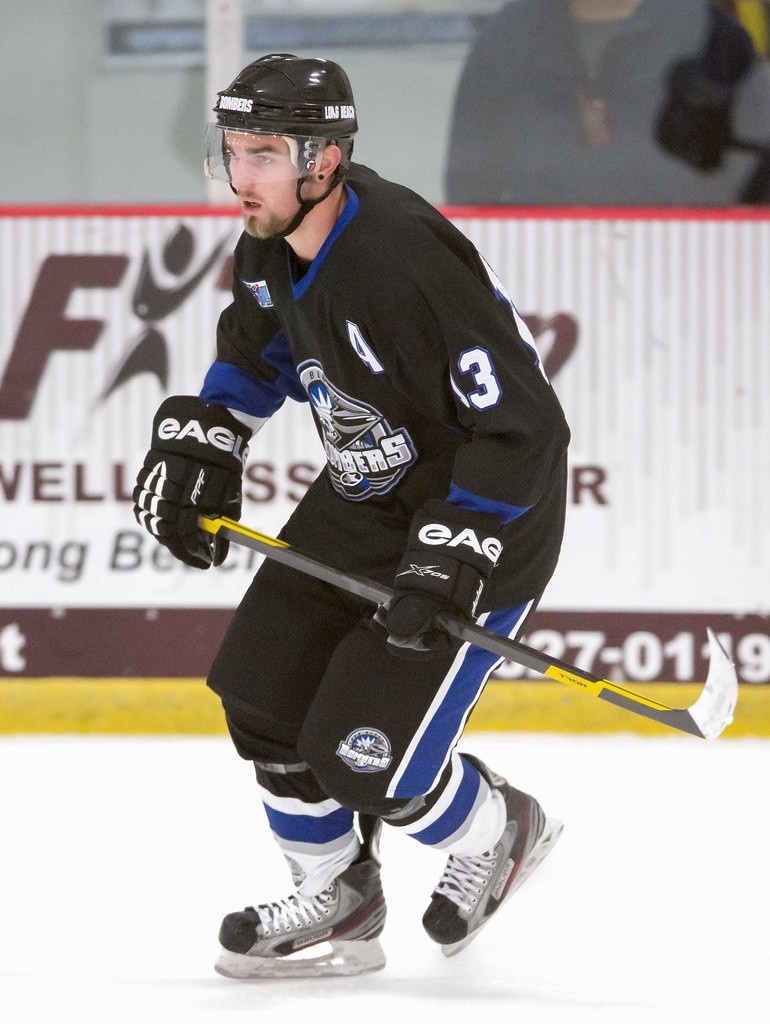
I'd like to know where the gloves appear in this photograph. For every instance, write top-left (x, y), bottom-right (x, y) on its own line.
top-left (132, 395), bottom-right (252, 568)
top-left (368, 500), bottom-right (508, 654)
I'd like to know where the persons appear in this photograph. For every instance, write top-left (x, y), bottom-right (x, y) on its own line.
top-left (446, 0), bottom-right (770, 204)
top-left (133, 54), bottom-right (570, 980)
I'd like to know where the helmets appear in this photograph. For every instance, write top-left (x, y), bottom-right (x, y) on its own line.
top-left (213, 54), bottom-right (359, 178)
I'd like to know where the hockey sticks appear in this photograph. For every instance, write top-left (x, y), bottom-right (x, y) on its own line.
top-left (196, 511), bottom-right (740, 742)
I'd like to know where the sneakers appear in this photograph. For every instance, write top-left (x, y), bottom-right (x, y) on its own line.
top-left (214, 858), bottom-right (386, 979)
top-left (422, 753), bottom-right (567, 957)
